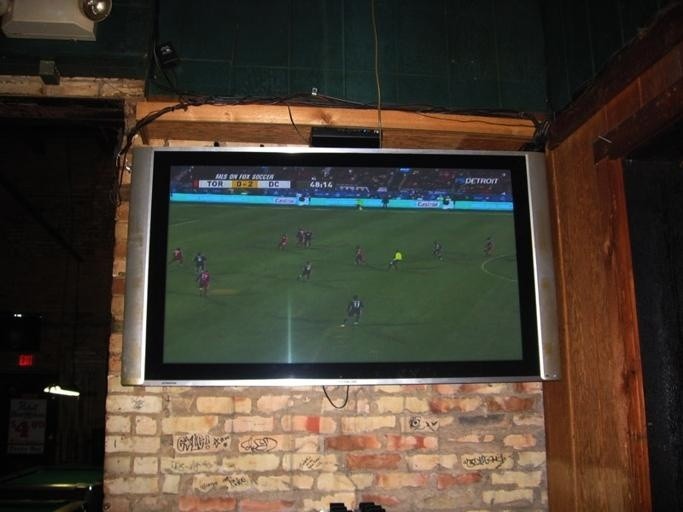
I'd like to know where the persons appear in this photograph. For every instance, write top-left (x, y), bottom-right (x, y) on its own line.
top-left (173, 247), bottom-right (184, 267)
top-left (352, 244), bottom-right (366, 265)
top-left (431, 239), bottom-right (446, 262)
top-left (480, 235), bottom-right (494, 256)
top-left (279, 231), bottom-right (288, 251)
top-left (190, 252), bottom-right (206, 273)
top-left (299, 260), bottom-right (312, 281)
top-left (192, 269), bottom-right (211, 297)
top-left (337, 293), bottom-right (364, 329)
top-left (387, 248), bottom-right (403, 272)
top-left (304, 227), bottom-right (312, 249)
top-left (294, 226), bottom-right (305, 248)
top-left (169, 166), bottom-right (513, 213)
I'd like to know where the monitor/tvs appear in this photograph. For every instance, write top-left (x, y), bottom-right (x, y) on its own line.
top-left (120, 145), bottom-right (564, 388)
